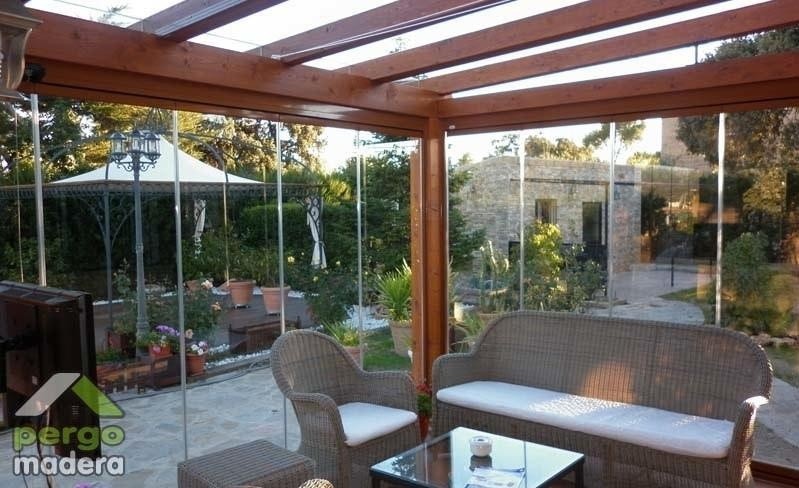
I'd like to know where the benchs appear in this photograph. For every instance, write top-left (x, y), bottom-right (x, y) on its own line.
top-left (428, 308), bottom-right (772, 486)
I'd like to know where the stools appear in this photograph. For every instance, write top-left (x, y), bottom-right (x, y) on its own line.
top-left (178, 438), bottom-right (315, 488)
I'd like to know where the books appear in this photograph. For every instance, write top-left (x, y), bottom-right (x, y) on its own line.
top-left (464, 466), bottom-right (527, 488)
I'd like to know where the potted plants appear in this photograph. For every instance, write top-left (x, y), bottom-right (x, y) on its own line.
top-left (374, 256), bottom-right (415, 357)
top-left (161, 223), bottom-right (292, 315)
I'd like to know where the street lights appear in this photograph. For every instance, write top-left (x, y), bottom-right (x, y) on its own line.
top-left (111, 127), bottom-right (160, 356)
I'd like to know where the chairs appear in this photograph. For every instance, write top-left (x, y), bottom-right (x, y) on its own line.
top-left (269, 329), bottom-right (419, 488)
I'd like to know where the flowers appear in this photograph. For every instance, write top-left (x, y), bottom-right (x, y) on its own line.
top-left (134, 324), bottom-right (211, 363)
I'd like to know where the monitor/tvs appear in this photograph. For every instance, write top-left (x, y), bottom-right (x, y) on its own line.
top-left (0, 276), bottom-right (104, 466)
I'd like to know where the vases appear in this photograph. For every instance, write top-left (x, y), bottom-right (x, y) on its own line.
top-left (184, 350), bottom-right (205, 376)
